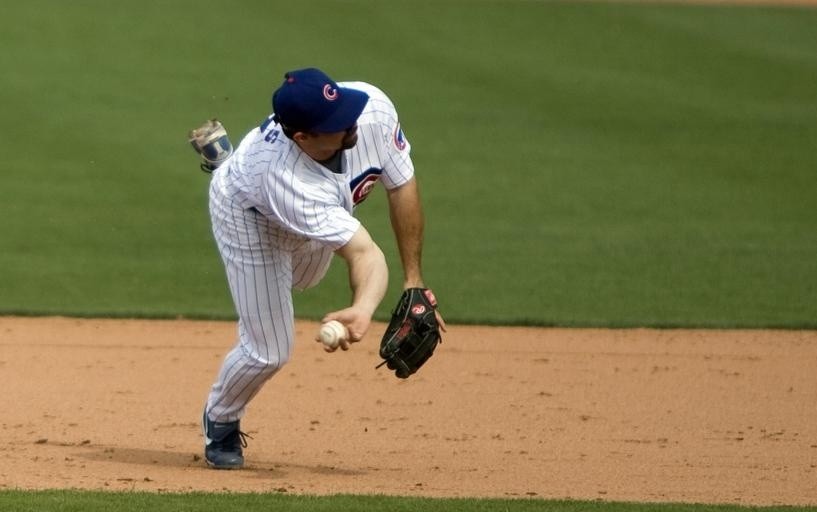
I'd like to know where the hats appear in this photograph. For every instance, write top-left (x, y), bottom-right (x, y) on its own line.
top-left (273, 67), bottom-right (369, 133)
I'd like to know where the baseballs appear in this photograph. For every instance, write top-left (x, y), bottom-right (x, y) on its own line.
top-left (319, 321), bottom-right (346, 348)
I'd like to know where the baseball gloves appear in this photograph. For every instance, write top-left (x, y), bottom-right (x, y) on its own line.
top-left (379, 289), bottom-right (442, 379)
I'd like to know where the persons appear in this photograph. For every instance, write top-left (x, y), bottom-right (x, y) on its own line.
top-left (187, 65), bottom-right (447, 471)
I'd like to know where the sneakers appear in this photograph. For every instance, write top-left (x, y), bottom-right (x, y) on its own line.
top-left (188, 120), bottom-right (234, 173)
top-left (202, 402), bottom-right (254, 469)
